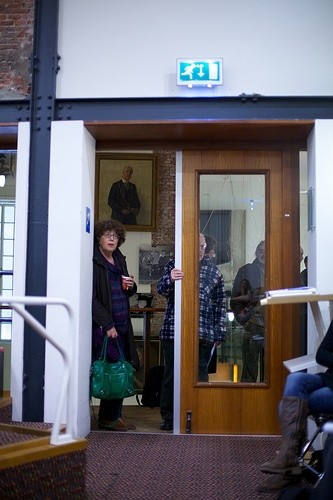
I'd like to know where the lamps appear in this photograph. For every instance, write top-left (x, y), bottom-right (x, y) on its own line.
top-left (0, 153), bottom-right (14, 187)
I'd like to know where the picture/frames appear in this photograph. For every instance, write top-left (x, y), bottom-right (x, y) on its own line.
top-left (133, 336), bottom-right (165, 394)
top-left (94, 152), bottom-right (158, 233)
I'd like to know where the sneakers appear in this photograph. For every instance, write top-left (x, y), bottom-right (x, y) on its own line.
top-left (120, 416), bottom-right (136, 430)
top-left (98, 417), bottom-right (129, 431)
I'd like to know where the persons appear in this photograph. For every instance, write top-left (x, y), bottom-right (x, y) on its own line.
top-left (155, 233), bottom-right (226, 433)
top-left (255, 319), bottom-right (332, 492)
top-left (107, 166), bottom-right (140, 224)
top-left (231, 240), bottom-right (266, 383)
top-left (92, 220), bottom-right (138, 431)
top-left (299, 244), bottom-right (309, 285)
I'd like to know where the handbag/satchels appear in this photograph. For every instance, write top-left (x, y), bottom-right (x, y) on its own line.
top-left (91, 334), bottom-right (135, 400)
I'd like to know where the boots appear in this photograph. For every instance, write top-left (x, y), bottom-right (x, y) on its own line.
top-left (256, 475), bottom-right (303, 492)
top-left (259, 397), bottom-right (310, 474)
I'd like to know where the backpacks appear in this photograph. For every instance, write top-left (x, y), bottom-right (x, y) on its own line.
top-left (141, 365), bottom-right (164, 406)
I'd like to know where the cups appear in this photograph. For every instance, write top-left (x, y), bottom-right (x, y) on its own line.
top-left (122, 277), bottom-right (132, 290)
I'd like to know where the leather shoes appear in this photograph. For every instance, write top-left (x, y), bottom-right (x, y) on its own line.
top-left (160, 415), bottom-right (173, 430)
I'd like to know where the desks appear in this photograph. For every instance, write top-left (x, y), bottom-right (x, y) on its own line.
top-left (130, 308), bottom-right (166, 385)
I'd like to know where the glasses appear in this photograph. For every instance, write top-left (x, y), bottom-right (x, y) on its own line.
top-left (199, 242), bottom-right (206, 250)
top-left (102, 233), bottom-right (119, 239)
